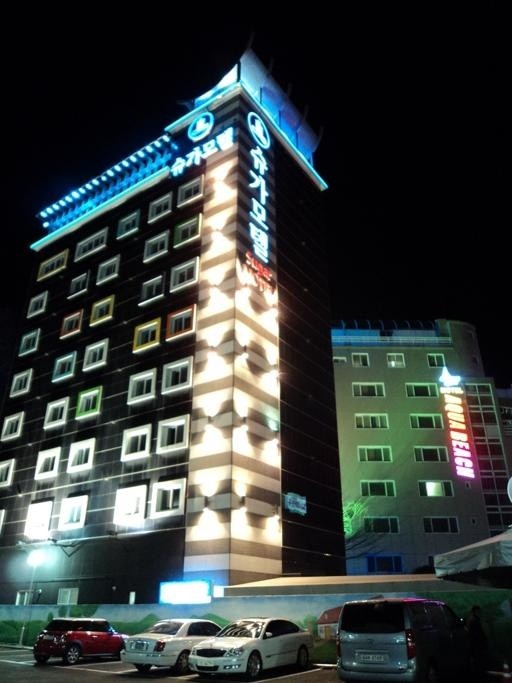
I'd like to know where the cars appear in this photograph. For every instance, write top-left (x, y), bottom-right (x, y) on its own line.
top-left (32, 615), bottom-right (128, 663)
top-left (188, 616), bottom-right (315, 681)
top-left (120, 617), bottom-right (223, 673)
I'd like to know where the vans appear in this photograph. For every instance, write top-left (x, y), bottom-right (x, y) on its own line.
top-left (334, 597), bottom-right (472, 682)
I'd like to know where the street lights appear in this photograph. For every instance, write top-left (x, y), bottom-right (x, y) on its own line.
top-left (16, 552), bottom-right (46, 648)
